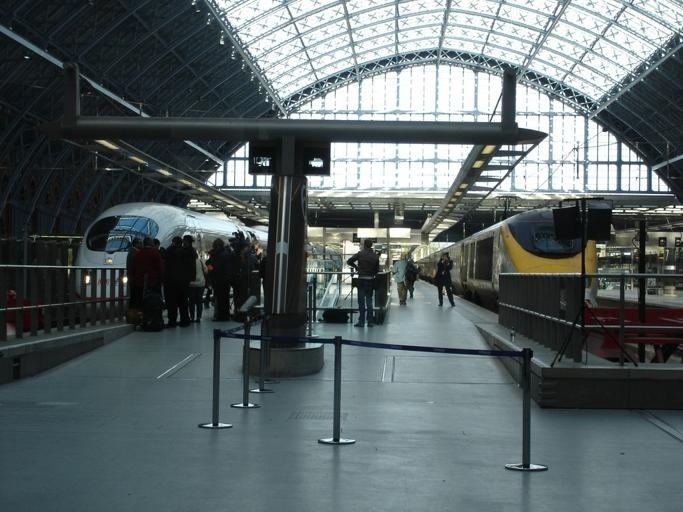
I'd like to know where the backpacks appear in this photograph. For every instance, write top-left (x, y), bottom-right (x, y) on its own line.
top-left (404, 260), bottom-right (417, 284)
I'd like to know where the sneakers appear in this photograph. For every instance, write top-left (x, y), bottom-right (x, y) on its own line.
top-left (400, 294), bottom-right (414, 306)
top-left (366, 324), bottom-right (373, 328)
top-left (353, 323), bottom-right (364, 327)
top-left (166, 317), bottom-right (201, 329)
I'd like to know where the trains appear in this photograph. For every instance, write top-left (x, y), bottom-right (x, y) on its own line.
top-left (74, 201), bottom-right (341, 315)
top-left (415, 206), bottom-right (598, 314)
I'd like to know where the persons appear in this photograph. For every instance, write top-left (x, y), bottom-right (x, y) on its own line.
top-left (346, 238), bottom-right (379, 327)
top-left (405, 257), bottom-right (416, 299)
top-left (432, 251), bottom-right (455, 307)
top-left (389, 252), bottom-right (418, 306)
top-left (126, 232), bottom-right (260, 333)
